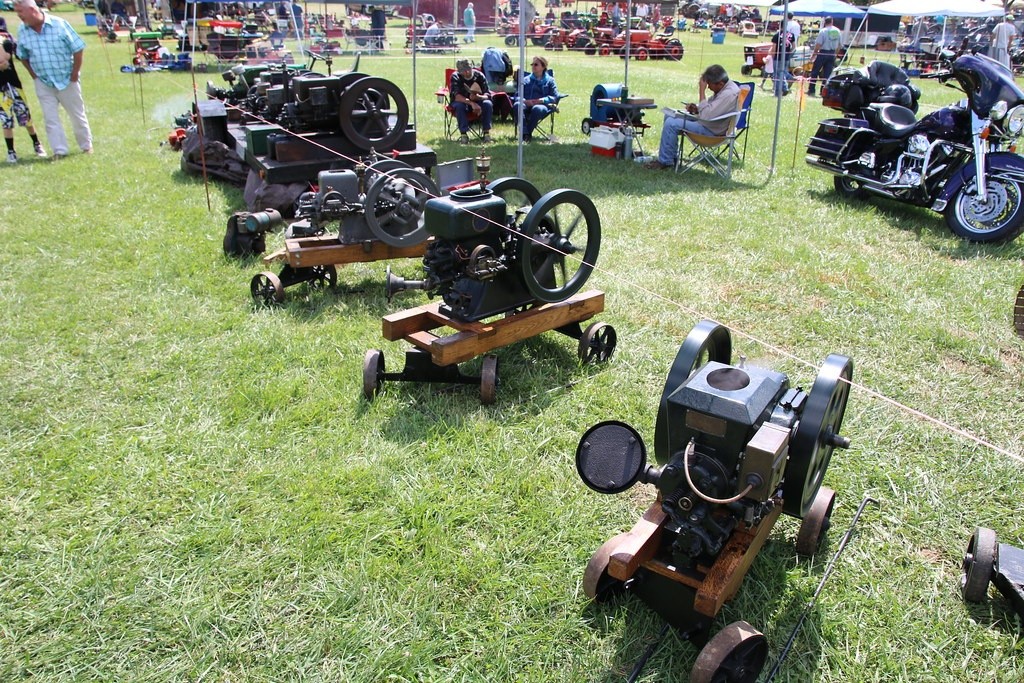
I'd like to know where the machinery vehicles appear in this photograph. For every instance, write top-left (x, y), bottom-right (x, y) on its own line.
top-left (504, 10), bottom-right (684, 61)
top-left (681, 0), bottom-right (723, 20)
top-left (741, 39), bottom-right (777, 78)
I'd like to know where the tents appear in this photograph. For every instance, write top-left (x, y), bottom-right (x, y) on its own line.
top-left (864, 0), bottom-right (1006, 73)
top-left (762, 0), bottom-right (868, 69)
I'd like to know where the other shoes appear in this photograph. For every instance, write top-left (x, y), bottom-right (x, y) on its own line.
top-left (8, 152), bottom-right (16, 163)
top-left (483, 136), bottom-right (493, 143)
top-left (523, 133), bottom-right (532, 144)
top-left (788, 77), bottom-right (796, 87)
top-left (804, 91), bottom-right (815, 96)
top-left (34, 143), bottom-right (46, 158)
top-left (53, 153), bottom-right (64, 160)
top-left (463, 38), bottom-right (468, 43)
top-left (783, 90), bottom-right (789, 95)
top-left (461, 136), bottom-right (468, 144)
top-left (645, 160), bottom-right (672, 169)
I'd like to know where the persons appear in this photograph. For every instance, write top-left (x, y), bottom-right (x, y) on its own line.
top-left (0, 17), bottom-right (47, 162)
top-left (609, 0), bottom-right (761, 32)
top-left (111, 0), bottom-right (304, 40)
top-left (423, 21), bottom-right (447, 46)
top-left (464, 2), bottom-right (477, 44)
top-left (483, 46), bottom-right (505, 85)
top-left (372, 6), bottom-right (387, 51)
top-left (14, 0), bottom-right (95, 159)
top-left (449, 58), bottom-right (494, 145)
top-left (643, 65), bottom-right (740, 171)
top-left (512, 56), bottom-right (559, 143)
top-left (757, 12), bottom-right (1016, 97)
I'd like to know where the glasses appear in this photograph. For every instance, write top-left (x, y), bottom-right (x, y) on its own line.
top-left (530, 63), bottom-right (542, 67)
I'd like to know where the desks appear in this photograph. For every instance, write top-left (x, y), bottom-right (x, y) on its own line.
top-left (598, 98), bottom-right (659, 160)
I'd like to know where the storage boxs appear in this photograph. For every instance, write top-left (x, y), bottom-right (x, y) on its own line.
top-left (591, 126), bottom-right (621, 156)
top-left (807, 119), bottom-right (869, 161)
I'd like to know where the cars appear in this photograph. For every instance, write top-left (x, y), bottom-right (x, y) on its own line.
top-left (899, 34), bottom-right (935, 53)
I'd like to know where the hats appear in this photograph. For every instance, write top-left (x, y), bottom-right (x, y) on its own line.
top-left (457, 59), bottom-right (472, 73)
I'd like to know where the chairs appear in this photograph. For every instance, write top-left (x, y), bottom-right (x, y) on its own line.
top-left (435, 66), bottom-right (568, 143)
top-left (671, 78), bottom-right (755, 179)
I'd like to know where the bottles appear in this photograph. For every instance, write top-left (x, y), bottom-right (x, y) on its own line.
top-left (633, 156), bottom-right (654, 163)
top-left (615, 142), bottom-right (625, 159)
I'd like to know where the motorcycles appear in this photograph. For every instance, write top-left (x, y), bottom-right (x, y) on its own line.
top-left (805, 25), bottom-right (1024, 244)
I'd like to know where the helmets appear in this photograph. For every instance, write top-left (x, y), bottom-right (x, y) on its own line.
top-left (885, 84), bottom-right (913, 109)
